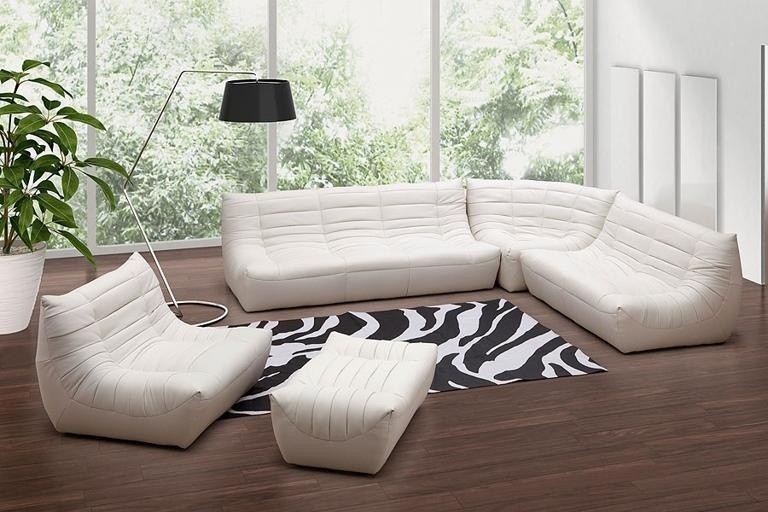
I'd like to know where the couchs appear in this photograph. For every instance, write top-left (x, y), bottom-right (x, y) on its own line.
top-left (466, 177), bottom-right (622, 294)
top-left (224, 179), bottom-right (504, 314)
top-left (520, 193), bottom-right (742, 353)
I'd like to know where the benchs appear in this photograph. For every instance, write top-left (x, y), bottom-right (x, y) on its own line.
top-left (268, 329), bottom-right (439, 477)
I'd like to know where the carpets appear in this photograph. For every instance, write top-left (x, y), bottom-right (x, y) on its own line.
top-left (218, 297), bottom-right (609, 422)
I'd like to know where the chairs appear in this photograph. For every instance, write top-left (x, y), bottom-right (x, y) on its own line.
top-left (34, 250), bottom-right (274, 450)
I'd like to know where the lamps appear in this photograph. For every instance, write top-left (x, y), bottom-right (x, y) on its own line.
top-left (122, 67), bottom-right (298, 326)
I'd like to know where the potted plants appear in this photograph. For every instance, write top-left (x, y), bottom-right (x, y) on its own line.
top-left (0, 51), bottom-right (134, 338)
